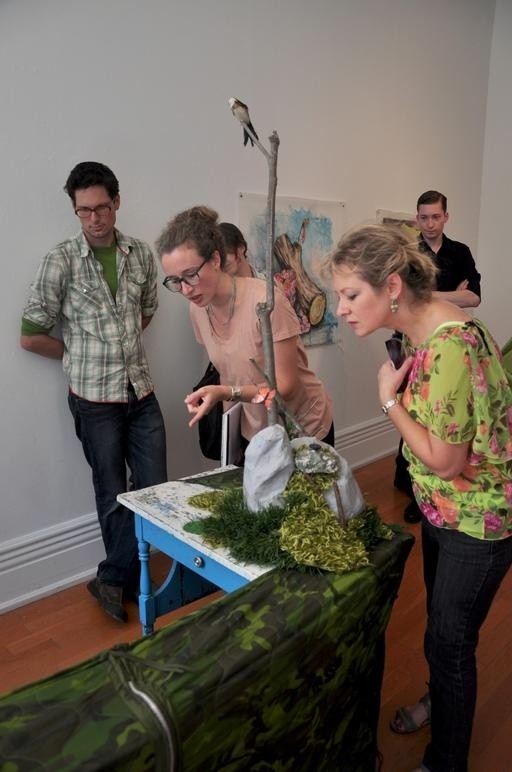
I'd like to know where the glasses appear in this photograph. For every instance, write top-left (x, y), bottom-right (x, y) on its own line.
top-left (74, 200), bottom-right (114, 218)
top-left (383, 339), bottom-right (408, 394)
top-left (162, 261), bottom-right (207, 293)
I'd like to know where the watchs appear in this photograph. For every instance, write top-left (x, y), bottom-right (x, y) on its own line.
top-left (380, 398), bottom-right (400, 416)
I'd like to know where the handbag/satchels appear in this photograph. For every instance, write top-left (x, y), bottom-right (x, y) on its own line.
top-left (192, 362), bottom-right (223, 462)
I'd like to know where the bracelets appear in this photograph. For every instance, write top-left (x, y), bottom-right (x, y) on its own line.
top-left (227, 384), bottom-right (242, 403)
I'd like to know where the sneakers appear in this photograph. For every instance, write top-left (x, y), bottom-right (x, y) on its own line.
top-left (86, 558), bottom-right (159, 623)
top-left (393, 478), bottom-right (423, 525)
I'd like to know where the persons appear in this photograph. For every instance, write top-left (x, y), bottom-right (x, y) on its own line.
top-left (215, 223), bottom-right (269, 279)
top-left (311, 223), bottom-right (509, 770)
top-left (396, 189), bottom-right (482, 523)
top-left (155, 204), bottom-right (335, 468)
top-left (21, 161), bottom-right (168, 624)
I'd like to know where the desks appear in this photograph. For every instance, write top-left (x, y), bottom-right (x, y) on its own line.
top-left (117, 462), bottom-right (283, 639)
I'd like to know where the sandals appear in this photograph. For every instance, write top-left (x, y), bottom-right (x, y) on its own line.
top-left (390, 690), bottom-right (429, 732)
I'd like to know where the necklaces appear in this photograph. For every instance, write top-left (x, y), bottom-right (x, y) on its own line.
top-left (209, 280), bottom-right (236, 324)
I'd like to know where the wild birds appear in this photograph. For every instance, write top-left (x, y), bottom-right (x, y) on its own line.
top-left (226, 95), bottom-right (260, 150)
